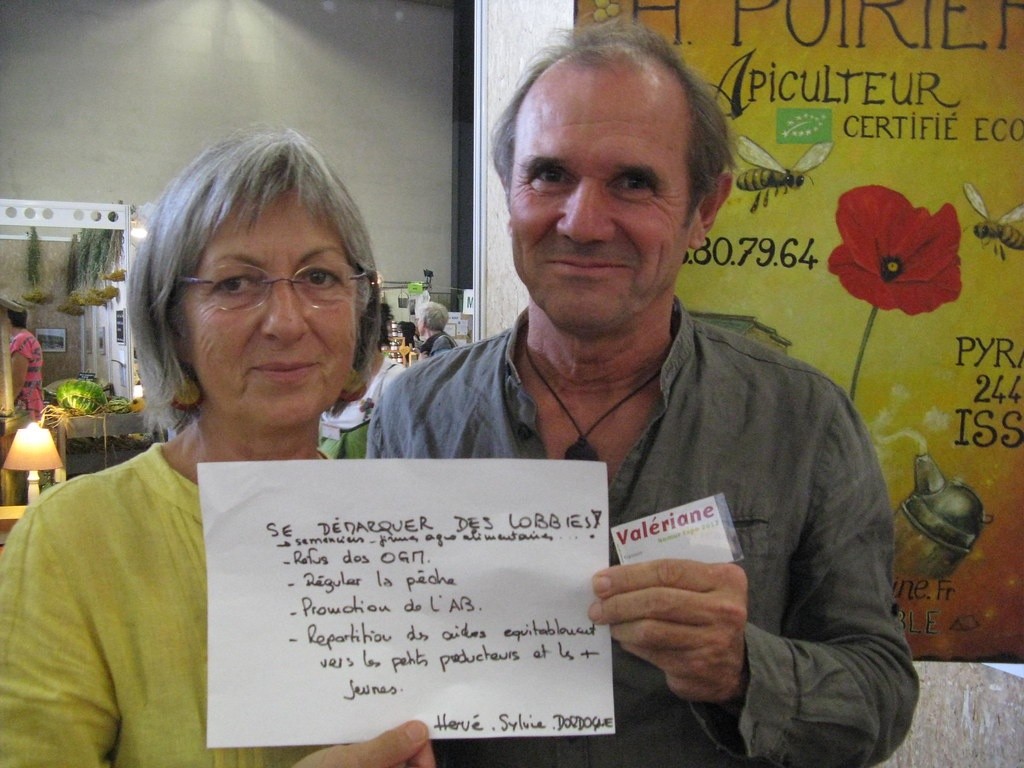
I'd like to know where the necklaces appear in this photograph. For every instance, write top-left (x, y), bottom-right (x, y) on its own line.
top-left (522, 332), bottom-right (659, 461)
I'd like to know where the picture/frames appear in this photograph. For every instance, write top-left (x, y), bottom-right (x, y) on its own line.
top-left (34, 327), bottom-right (68, 352)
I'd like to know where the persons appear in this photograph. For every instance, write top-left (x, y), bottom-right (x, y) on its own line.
top-left (0, 129), bottom-right (456, 768)
top-left (359, 16), bottom-right (920, 768)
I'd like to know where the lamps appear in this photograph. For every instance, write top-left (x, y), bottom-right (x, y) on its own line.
top-left (4, 420), bottom-right (65, 510)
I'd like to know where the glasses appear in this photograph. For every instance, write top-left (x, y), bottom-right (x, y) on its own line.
top-left (175, 261), bottom-right (369, 311)
